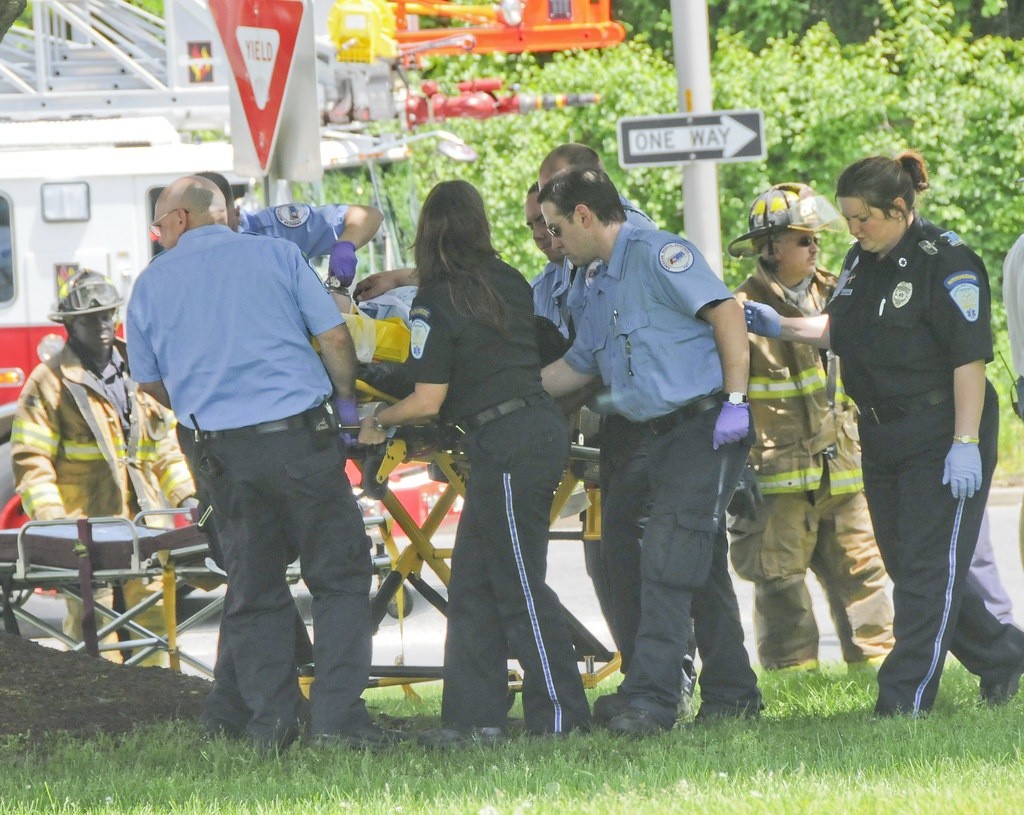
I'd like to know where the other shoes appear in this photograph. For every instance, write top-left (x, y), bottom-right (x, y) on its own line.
top-left (593, 693), bottom-right (630, 720)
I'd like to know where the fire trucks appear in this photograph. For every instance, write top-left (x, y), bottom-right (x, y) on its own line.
top-left (1, 2), bottom-right (627, 595)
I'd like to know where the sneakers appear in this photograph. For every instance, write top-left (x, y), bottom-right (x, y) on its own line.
top-left (980, 665), bottom-right (1024, 708)
top-left (608, 699), bottom-right (670, 738)
top-left (678, 711), bottom-right (760, 732)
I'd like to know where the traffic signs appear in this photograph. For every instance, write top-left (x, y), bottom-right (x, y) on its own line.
top-left (618, 109), bottom-right (767, 171)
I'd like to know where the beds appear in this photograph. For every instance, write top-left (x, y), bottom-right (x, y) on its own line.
top-left (0, 506), bottom-right (393, 679)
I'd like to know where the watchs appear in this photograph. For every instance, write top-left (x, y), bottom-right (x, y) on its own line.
top-left (722, 392), bottom-right (749, 406)
top-left (371, 416), bottom-right (392, 436)
top-left (952, 434), bottom-right (979, 447)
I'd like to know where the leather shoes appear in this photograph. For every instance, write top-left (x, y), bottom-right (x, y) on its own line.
top-left (417, 726), bottom-right (508, 753)
top-left (309, 719), bottom-right (411, 753)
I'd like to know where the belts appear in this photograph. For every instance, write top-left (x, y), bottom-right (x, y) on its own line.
top-left (646, 390), bottom-right (724, 438)
top-left (855, 382), bottom-right (954, 425)
top-left (453, 390), bottom-right (552, 435)
top-left (176, 406), bottom-right (328, 441)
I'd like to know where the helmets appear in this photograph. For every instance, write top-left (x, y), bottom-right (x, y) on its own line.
top-left (727, 182), bottom-right (845, 259)
top-left (47, 268), bottom-right (127, 322)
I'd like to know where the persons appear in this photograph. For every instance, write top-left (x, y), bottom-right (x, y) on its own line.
top-left (1002, 232), bottom-right (1024, 578)
top-left (525, 143), bottom-right (765, 739)
top-left (743, 152), bottom-right (1024, 720)
top-left (11, 270), bottom-right (200, 668)
top-left (127, 172), bottom-right (411, 754)
top-left (359, 180), bottom-right (594, 749)
top-left (727, 182), bottom-right (896, 683)
top-left (309, 266), bottom-right (572, 418)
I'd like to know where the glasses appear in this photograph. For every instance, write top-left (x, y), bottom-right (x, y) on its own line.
top-left (800, 236), bottom-right (820, 246)
top-left (148, 208), bottom-right (189, 237)
top-left (57, 282), bottom-right (121, 311)
top-left (546, 210), bottom-right (574, 237)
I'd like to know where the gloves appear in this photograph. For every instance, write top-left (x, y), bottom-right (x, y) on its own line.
top-left (181, 497), bottom-right (200, 521)
top-left (942, 444), bottom-right (983, 501)
top-left (328, 241), bottom-right (358, 287)
top-left (712, 402), bottom-right (750, 450)
top-left (727, 467), bottom-right (764, 521)
top-left (332, 399), bottom-right (366, 450)
top-left (742, 300), bottom-right (782, 339)
